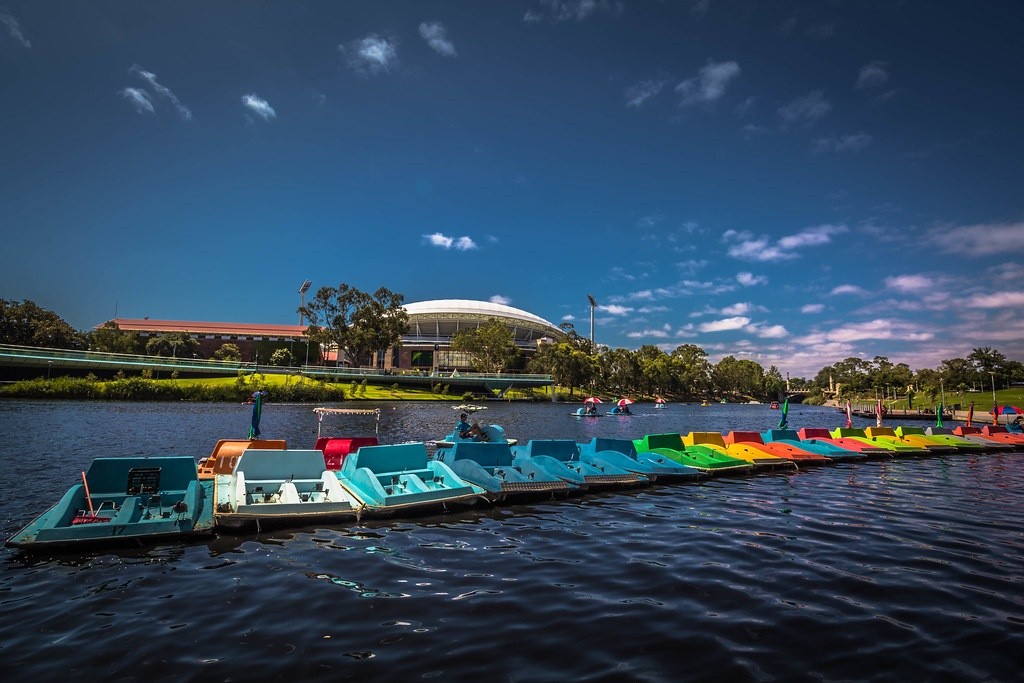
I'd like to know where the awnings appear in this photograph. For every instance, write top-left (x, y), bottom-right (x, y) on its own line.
top-left (989, 405), bottom-right (1024, 423)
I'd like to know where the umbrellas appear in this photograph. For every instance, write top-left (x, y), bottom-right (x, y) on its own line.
top-left (965, 402), bottom-right (974, 426)
top-left (876, 400), bottom-right (883, 428)
top-left (846, 401), bottom-right (852, 428)
top-left (586, 396), bottom-right (604, 404)
top-left (617, 399), bottom-right (635, 406)
top-left (247, 389), bottom-right (263, 439)
top-left (778, 398), bottom-right (789, 430)
top-left (935, 403), bottom-right (943, 428)
top-left (993, 401), bottom-right (998, 426)
top-left (655, 398), bottom-right (666, 403)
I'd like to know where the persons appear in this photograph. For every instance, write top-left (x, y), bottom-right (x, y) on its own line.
top-left (587, 404), bottom-right (597, 414)
top-left (619, 404), bottom-right (629, 413)
top-left (457, 413), bottom-right (488, 441)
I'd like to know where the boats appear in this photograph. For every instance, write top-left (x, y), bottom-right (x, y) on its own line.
top-left (741, 401), bottom-right (762, 404)
top-left (769, 401), bottom-right (780, 409)
top-left (571, 408), bottom-right (603, 416)
top-left (195, 438), bottom-right (288, 481)
top-left (701, 402), bottom-right (712, 406)
top-left (4, 425), bottom-right (1024, 549)
top-left (654, 404), bottom-right (667, 408)
top-left (721, 402), bottom-right (727, 404)
top-left (433, 422), bottom-right (518, 449)
top-left (313, 434), bottom-right (380, 471)
top-left (465, 404), bottom-right (477, 412)
top-left (606, 407), bottom-right (633, 415)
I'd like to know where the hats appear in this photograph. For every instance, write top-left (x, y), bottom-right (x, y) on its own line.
top-left (460, 413), bottom-right (468, 418)
top-left (470, 423), bottom-right (480, 429)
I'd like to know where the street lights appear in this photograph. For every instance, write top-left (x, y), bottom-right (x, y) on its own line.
top-left (987, 372), bottom-right (998, 406)
top-left (874, 383), bottom-right (898, 403)
top-left (585, 294), bottom-right (598, 343)
